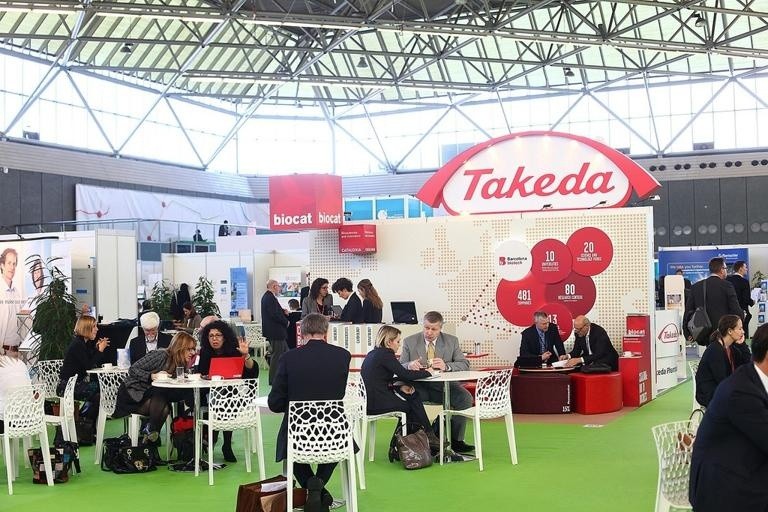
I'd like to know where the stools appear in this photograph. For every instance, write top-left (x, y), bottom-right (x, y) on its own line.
top-left (568, 372), bottom-right (624, 415)
top-left (510, 372), bottom-right (574, 414)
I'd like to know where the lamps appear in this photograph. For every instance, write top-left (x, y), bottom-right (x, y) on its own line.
top-left (692, 14), bottom-right (706, 27)
top-left (563, 68), bottom-right (574, 76)
top-left (357, 57), bottom-right (368, 67)
top-left (120, 43), bottom-right (133, 52)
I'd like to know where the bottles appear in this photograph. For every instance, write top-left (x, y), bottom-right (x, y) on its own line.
top-left (476, 342), bottom-right (481, 356)
top-left (541, 362), bottom-right (547, 369)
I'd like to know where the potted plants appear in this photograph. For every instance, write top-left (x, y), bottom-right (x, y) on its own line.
top-left (18, 253), bottom-right (91, 424)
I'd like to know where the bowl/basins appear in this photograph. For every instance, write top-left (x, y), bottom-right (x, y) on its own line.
top-left (188, 373), bottom-right (201, 382)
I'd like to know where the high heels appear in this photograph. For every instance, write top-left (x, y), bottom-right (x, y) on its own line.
top-left (142, 424), bottom-right (159, 444)
top-left (155, 448), bottom-right (167, 466)
top-left (388, 448), bottom-right (400, 462)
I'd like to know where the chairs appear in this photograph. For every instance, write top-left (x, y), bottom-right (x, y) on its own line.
top-left (0, 318), bottom-right (270, 495)
top-left (651, 420), bottom-right (700, 512)
top-left (687, 360), bottom-right (707, 428)
top-left (438, 368), bottom-right (518, 471)
top-left (283, 372), bottom-right (407, 512)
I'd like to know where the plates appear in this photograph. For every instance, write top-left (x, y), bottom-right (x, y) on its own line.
top-left (99, 366), bottom-right (116, 370)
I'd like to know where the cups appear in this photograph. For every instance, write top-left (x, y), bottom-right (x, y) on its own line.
top-left (116, 347), bottom-right (131, 369)
top-left (102, 362), bottom-right (113, 369)
top-left (211, 375), bottom-right (226, 382)
top-left (176, 366), bottom-right (185, 384)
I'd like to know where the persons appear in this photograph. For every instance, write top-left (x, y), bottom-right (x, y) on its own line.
top-left (19, 258), bottom-right (56, 350)
top-left (398, 311), bottom-right (476, 453)
top-left (138, 299), bottom-right (153, 326)
top-left (169, 283), bottom-right (190, 318)
top-left (123, 312), bottom-right (173, 367)
top-left (285, 298), bottom-right (301, 349)
top-left (301, 278), bottom-right (382, 324)
top-left (520, 312), bottom-right (565, 368)
top-left (266, 314), bottom-right (360, 511)
top-left (112, 332), bottom-right (198, 458)
top-left (682, 257), bottom-right (747, 347)
top-left (559, 314), bottom-right (618, 372)
top-left (689, 322), bottom-right (767, 511)
top-left (217, 220), bottom-right (230, 235)
top-left (725, 261), bottom-right (756, 334)
top-left (57, 316), bottom-right (110, 448)
top-left (193, 230), bottom-right (202, 241)
top-left (195, 320), bottom-right (259, 464)
top-left (0, 247), bottom-right (24, 361)
top-left (361, 325), bottom-right (449, 464)
top-left (182, 301), bottom-right (202, 332)
top-left (695, 314), bottom-right (751, 408)
top-left (33, 280), bottom-right (79, 362)
top-left (261, 279), bottom-right (291, 387)
top-left (675, 269), bottom-right (690, 288)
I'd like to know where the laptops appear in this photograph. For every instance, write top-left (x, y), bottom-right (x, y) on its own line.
top-left (331, 305), bottom-right (342, 315)
top-left (200, 357), bottom-right (245, 380)
top-left (391, 302), bottom-right (418, 324)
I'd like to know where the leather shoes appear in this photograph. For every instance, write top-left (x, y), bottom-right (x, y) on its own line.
top-left (222, 445), bottom-right (237, 462)
top-left (425, 431), bottom-right (474, 452)
top-left (304, 477), bottom-right (332, 512)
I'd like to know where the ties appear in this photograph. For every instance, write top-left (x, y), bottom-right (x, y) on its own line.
top-left (427, 342), bottom-right (434, 360)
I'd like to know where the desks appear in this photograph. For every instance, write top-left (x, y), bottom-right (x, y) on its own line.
top-left (518, 368), bottom-right (575, 372)
top-left (411, 371), bottom-right (492, 464)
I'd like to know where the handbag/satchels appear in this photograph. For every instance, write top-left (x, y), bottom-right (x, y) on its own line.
top-left (28, 441), bottom-right (79, 485)
top-left (394, 422), bottom-right (431, 470)
top-left (686, 307), bottom-right (711, 340)
top-left (52, 421), bottom-right (93, 446)
top-left (103, 434), bottom-right (156, 473)
top-left (170, 416), bottom-right (207, 460)
top-left (580, 363), bottom-right (611, 373)
top-left (676, 433), bottom-right (694, 465)
top-left (235, 476), bottom-right (305, 512)
